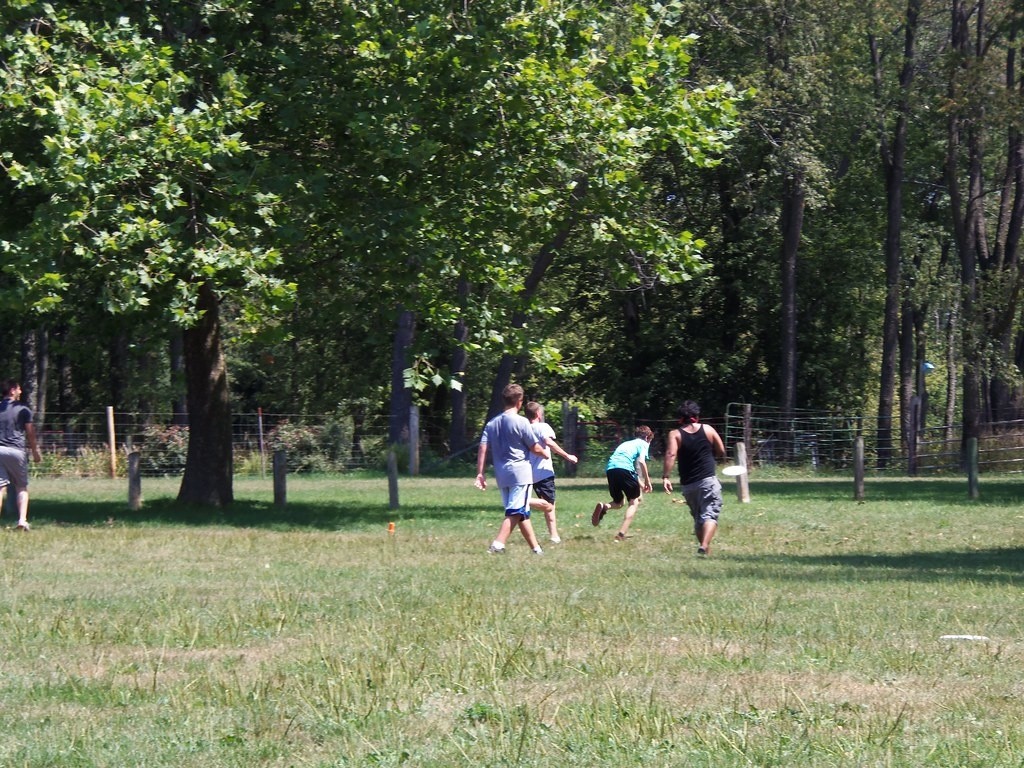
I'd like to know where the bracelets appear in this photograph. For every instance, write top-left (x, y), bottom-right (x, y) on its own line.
top-left (663, 475), bottom-right (669, 479)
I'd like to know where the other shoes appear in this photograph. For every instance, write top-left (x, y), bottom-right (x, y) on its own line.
top-left (17, 520), bottom-right (29, 531)
top-left (698, 546), bottom-right (706, 554)
top-left (487, 546), bottom-right (505, 554)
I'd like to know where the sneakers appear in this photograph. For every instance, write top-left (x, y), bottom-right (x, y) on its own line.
top-left (614, 535), bottom-right (625, 542)
top-left (592, 502), bottom-right (606, 526)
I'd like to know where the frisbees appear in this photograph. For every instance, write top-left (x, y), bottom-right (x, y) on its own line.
top-left (721, 466), bottom-right (747, 476)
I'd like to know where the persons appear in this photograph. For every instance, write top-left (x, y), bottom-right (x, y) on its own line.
top-left (0, 377), bottom-right (41, 532)
top-left (663, 402), bottom-right (725, 556)
top-left (591, 426), bottom-right (654, 542)
top-left (474, 383), bottom-right (549, 555)
top-left (524, 401), bottom-right (578, 544)
